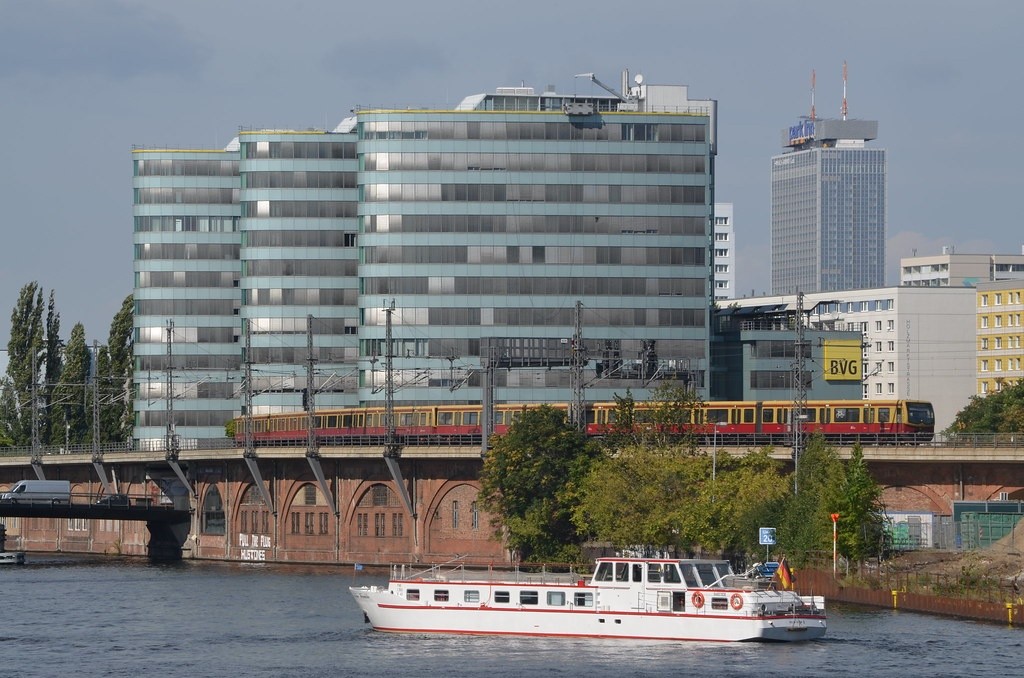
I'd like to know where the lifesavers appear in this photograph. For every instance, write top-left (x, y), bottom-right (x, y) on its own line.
top-left (691, 592), bottom-right (705, 608)
top-left (729, 592), bottom-right (744, 611)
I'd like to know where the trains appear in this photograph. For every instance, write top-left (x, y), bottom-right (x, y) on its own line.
top-left (233, 399), bottom-right (935, 448)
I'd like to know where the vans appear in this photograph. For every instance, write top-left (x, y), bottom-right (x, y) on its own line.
top-left (0, 480), bottom-right (71, 505)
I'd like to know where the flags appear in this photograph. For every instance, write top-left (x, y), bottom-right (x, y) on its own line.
top-left (777, 559), bottom-right (796, 590)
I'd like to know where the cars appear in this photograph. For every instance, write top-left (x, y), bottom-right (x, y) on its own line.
top-left (94, 494), bottom-right (131, 506)
top-left (745, 562), bottom-right (780, 580)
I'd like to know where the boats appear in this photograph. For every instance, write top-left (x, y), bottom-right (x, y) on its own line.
top-left (0, 524), bottom-right (26, 564)
top-left (348, 553), bottom-right (827, 642)
